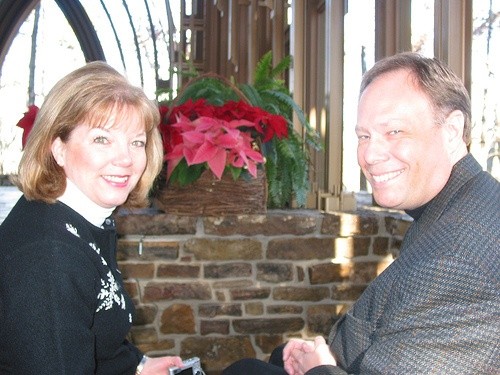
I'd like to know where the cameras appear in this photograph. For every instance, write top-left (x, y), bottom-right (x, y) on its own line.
top-left (168, 356), bottom-right (202, 375)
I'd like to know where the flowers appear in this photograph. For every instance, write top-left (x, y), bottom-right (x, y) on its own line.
top-left (152, 49), bottom-right (326, 210)
top-left (16, 103), bottom-right (43, 154)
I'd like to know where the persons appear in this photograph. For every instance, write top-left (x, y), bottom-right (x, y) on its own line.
top-left (221, 51), bottom-right (500, 375)
top-left (0, 60), bottom-right (185, 375)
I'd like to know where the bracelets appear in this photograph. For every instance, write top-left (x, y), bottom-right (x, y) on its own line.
top-left (135, 354), bottom-right (148, 375)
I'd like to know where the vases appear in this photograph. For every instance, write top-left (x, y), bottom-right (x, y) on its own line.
top-left (157, 71), bottom-right (268, 216)
